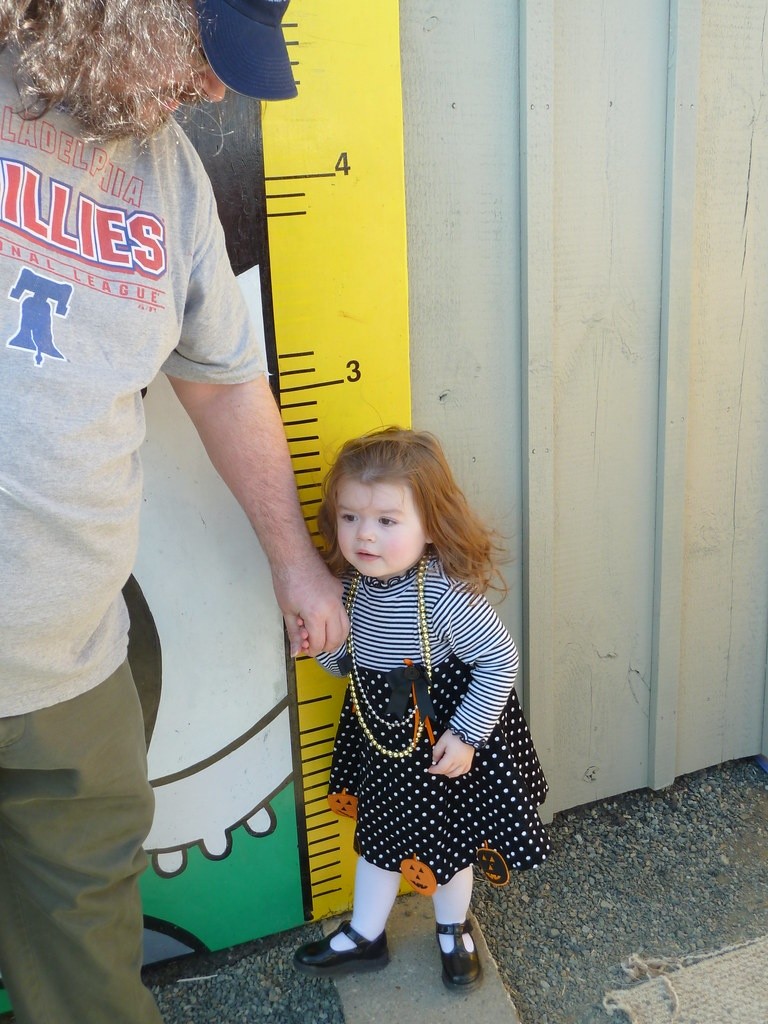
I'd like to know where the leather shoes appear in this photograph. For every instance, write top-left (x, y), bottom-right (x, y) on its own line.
top-left (435, 917), bottom-right (483, 995)
top-left (292, 920), bottom-right (390, 978)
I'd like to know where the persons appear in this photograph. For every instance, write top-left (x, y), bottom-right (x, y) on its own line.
top-left (292, 427), bottom-right (550, 993)
top-left (0, 0), bottom-right (349, 1024)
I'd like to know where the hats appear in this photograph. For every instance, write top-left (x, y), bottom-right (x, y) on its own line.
top-left (194, 1), bottom-right (300, 103)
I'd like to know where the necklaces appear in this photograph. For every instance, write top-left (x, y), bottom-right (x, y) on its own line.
top-left (343, 550), bottom-right (431, 757)
